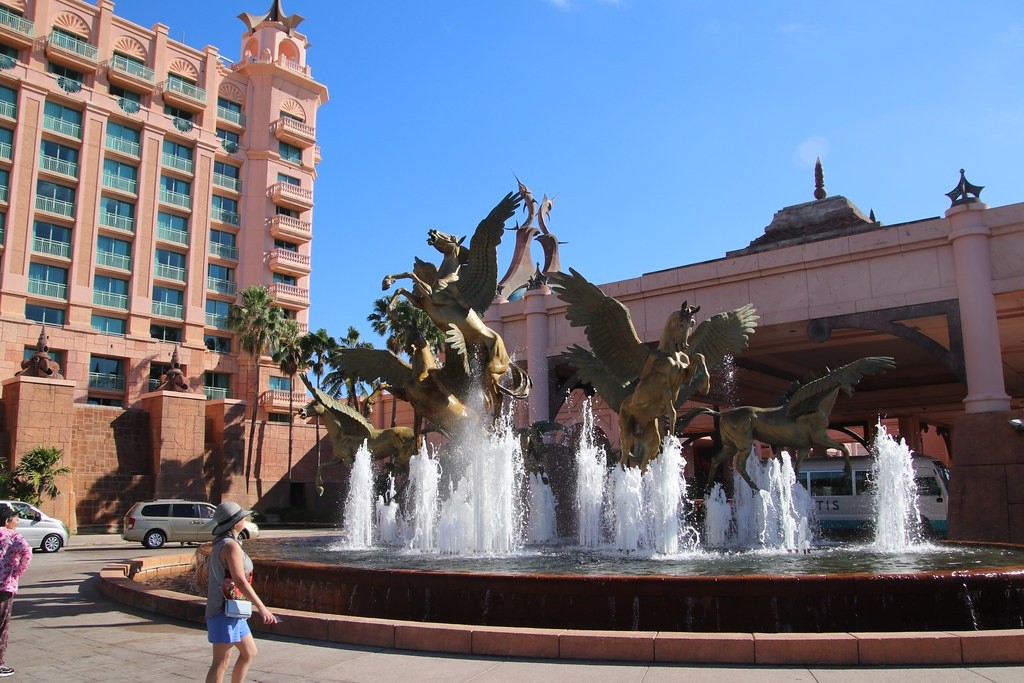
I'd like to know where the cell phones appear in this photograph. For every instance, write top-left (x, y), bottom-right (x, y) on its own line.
top-left (273, 616), bottom-right (283, 624)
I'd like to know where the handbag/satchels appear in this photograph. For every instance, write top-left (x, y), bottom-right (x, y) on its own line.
top-left (225, 599), bottom-right (253, 619)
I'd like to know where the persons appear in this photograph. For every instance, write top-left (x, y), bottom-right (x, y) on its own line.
top-left (205, 501), bottom-right (277, 683)
top-left (0, 509), bottom-right (32, 677)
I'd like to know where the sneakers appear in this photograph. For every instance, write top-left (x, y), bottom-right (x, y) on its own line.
top-left (0, 666), bottom-right (15, 678)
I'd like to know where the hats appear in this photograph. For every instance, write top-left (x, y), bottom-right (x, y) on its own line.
top-left (211, 501), bottom-right (255, 536)
top-left (0, 503), bottom-right (24, 522)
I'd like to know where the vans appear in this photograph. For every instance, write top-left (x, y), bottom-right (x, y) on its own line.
top-left (122, 499), bottom-right (258, 549)
top-left (755, 454), bottom-right (947, 539)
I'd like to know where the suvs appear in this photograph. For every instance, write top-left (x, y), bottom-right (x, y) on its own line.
top-left (0, 500), bottom-right (68, 552)
